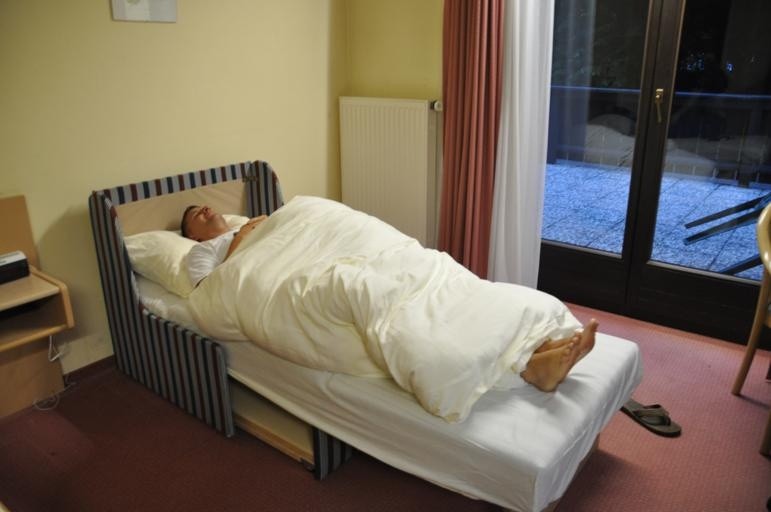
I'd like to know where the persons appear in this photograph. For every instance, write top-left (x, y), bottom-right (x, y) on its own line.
top-left (181, 204), bottom-right (599, 392)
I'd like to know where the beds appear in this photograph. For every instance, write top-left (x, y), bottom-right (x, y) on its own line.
top-left (86, 156), bottom-right (641, 510)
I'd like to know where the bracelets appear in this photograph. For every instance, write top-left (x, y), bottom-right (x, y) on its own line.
top-left (232, 231), bottom-right (243, 240)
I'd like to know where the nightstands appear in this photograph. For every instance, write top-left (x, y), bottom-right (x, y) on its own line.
top-left (1, 194), bottom-right (75, 434)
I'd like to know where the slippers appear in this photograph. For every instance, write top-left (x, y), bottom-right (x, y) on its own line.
top-left (622, 396), bottom-right (682, 438)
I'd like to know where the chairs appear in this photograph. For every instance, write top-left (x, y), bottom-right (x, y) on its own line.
top-left (727, 194), bottom-right (771, 453)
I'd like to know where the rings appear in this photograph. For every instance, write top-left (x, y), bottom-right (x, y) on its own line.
top-left (252, 226), bottom-right (255, 229)
top-left (247, 223), bottom-right (250, 225)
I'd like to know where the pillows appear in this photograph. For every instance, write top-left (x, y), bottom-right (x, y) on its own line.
top-left (116, 210), bottom-right (248, 294)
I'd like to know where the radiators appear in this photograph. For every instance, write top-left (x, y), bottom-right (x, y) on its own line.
top-left (337, 93), bottom-right (443, 247)
